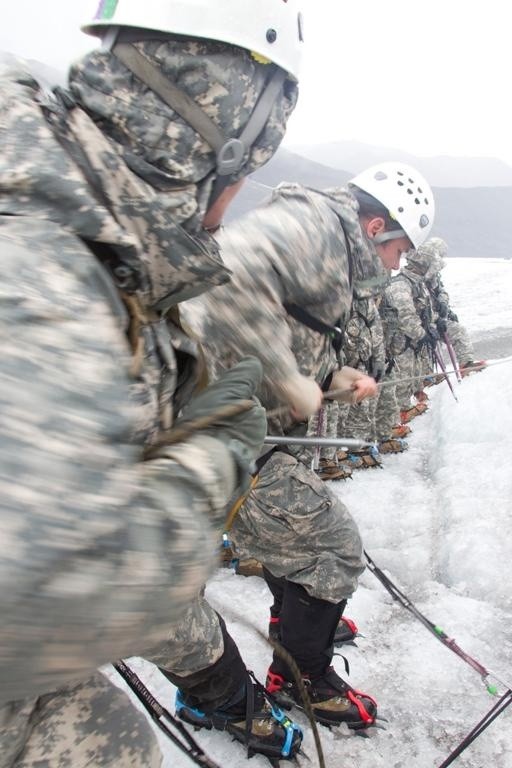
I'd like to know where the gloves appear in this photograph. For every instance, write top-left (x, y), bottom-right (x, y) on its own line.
top-left (417, 303), bottom-right (461, 348)
top-left (175, 354), bottom-right (268, 476)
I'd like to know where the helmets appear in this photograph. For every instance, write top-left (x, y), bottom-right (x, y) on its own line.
top-left (406, 237), bottom-right (449, 267)
top-left (347, 160), bottom-right (436, 252)
top-left (78, 0), bottom-right (310, 90)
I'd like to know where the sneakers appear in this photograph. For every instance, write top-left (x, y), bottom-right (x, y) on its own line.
top-left (172, 688), bottom-right (303, 761)
top-left (317, 370), bottom-right (449, 481)
top-left (459, 360), bottom-right (487, 379)
top-left (260, 608), bottom-right (360, 650)
top-left (266, 660), bottom-right (378, 730)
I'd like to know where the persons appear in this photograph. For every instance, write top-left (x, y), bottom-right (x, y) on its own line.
top-left (177, 159), bottom-right (439, 727)
top-left (1, 0), bottom-right (305, 768)
